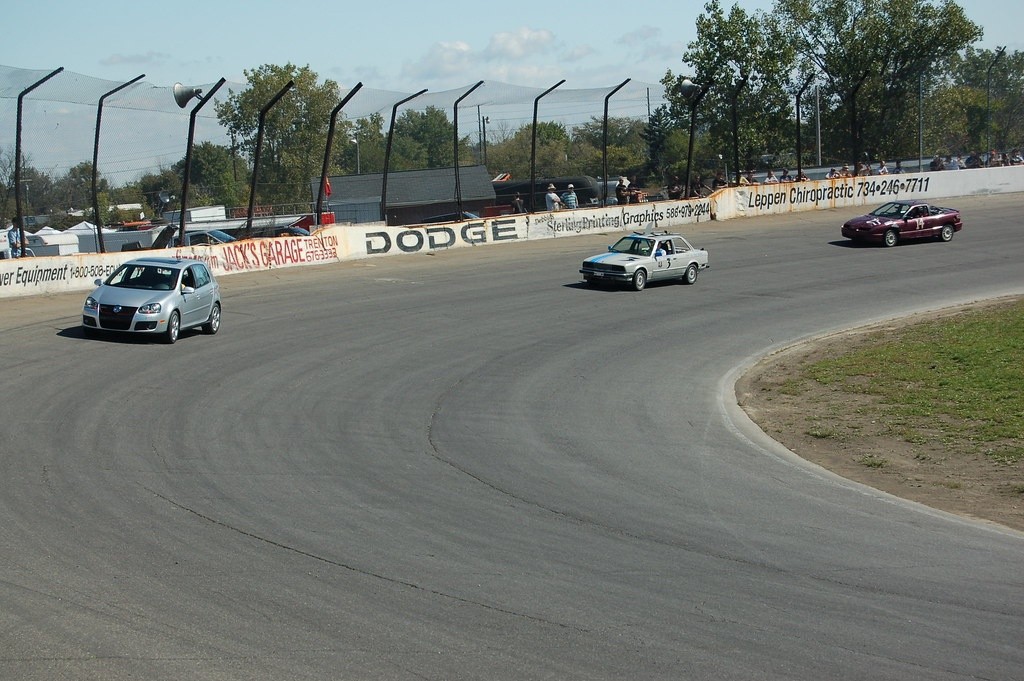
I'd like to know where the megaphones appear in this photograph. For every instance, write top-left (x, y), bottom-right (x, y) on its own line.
top-left (172, 82), bottom-right (202, 108)
top-left (680, 78), bottom-right (701, 97)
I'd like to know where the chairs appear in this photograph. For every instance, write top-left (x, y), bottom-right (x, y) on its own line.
top-left (662, 244), bottom-right (668, 254)
top-left (139, 271), bottom-right (166, 285)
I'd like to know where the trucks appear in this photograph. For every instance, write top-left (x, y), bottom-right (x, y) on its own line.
top-left (167, 229), bottom-right (235, 249)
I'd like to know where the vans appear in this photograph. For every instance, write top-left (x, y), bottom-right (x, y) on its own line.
top-left (235, 224), bottom-right (311, 245)
top-left (421, 211), bottom-right (480, 223)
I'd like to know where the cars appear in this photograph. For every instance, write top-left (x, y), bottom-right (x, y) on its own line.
top-left (82, 256), bottom-right (222, 344)
top-left (841, 200), bottom-right (963, 247)
top-left (579, 230), bottom-right (710, 291)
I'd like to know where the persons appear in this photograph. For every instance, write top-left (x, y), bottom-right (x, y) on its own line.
top-left (545, 183), bottom-right (561, 211)
top-left (559, 184), bottom-right (578, 209)
top-left (795, 169), bottom-right (810, 181)
top-left (930, 148), bottom-right (1024, 171)
top-left (690, 173), bottom-right (714, 198)
top-left (909, 207), bottom-right (919, 218)
top-left (712, 171), bottom-right (728, 191)
top-left (840, 160), bottom-right (906, 178)
top-left (649, 241), bottom-right (666, 256)
top-left (511, 191), bottom-right (523, 214)
top-left (780, 169), bottom-right (794, 183)
top-left (667, 175), bottom-right (683, 200)
top-left (764, 171), bottom-right (779, 185)
top-left (826, 168), bottom-right (840, 179)
top-left (729, 170), bottom-right (749, 186)
top-left (167, 269), bottom-right (185, 290)
top-left (746, 170), bottom-right (760, 186)
top-left (627, 175), bottom-right (641, 203)
top-left (8, 217), bottom-right (29, 258)
top-left (616, 176), bottom-right (630, 205)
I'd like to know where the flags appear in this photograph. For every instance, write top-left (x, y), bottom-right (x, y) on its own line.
top-left (325, 176), bottom-right (331, 196)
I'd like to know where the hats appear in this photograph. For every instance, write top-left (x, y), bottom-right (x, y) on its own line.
top-left (568, 184), bottom-right (574, 188)
top-left (547, 184), bottom-right (556, 190)
